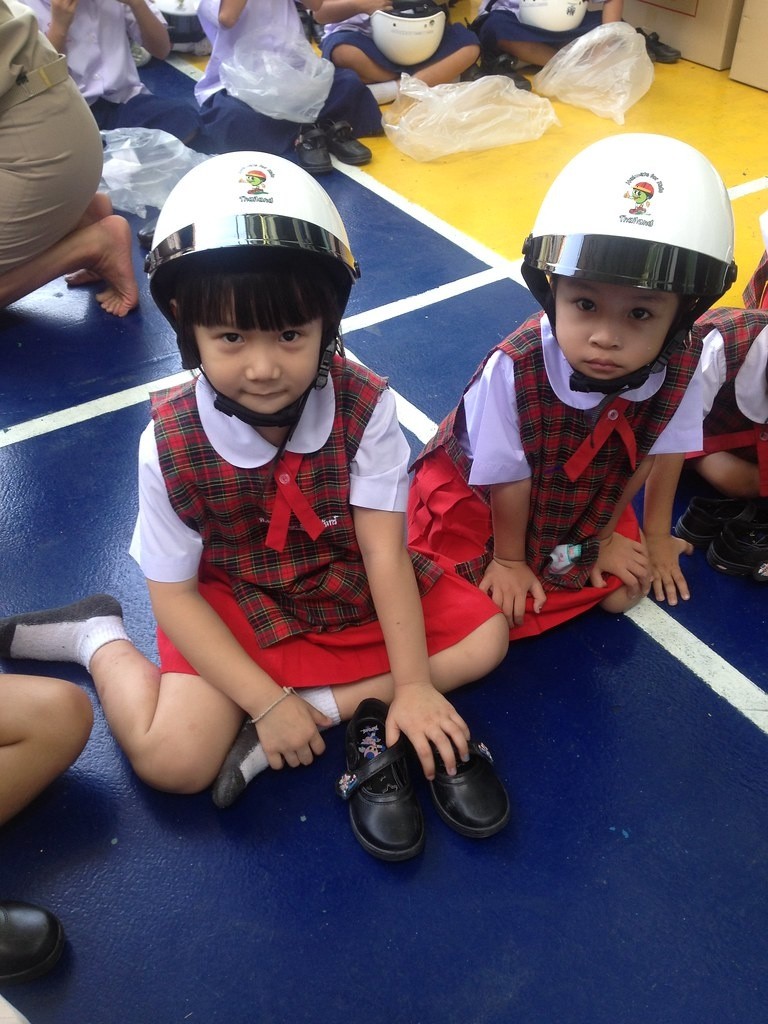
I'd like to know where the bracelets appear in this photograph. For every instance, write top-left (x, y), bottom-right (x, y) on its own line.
top-left (252, 686), bottom-right (296, 726)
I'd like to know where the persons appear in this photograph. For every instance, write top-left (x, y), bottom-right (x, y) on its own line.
top-left (406, 132), bottom-right (768, 640)
top-left (1, 150), bottom-right (510, 808)
top-left (0, 0), bottom-right (139, 317)
top-left (0, 674), bottom-right (94, 1024)
top-left (0, 0), bottom-right (624, 150)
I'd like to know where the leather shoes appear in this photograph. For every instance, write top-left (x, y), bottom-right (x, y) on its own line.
top-left (0, 905), bottom-right (63, 986)
top-left (296, 124), bottom-right (332, 172)
top-left (428, 735), bottom-right (508, 841)
top-left (706, 520), bottom-right (768, 582)
top-left (674, 496), bottom-right (768, 544)
top-left (317, 120), bottom-right (372, 164)
top-left (337, 696), bottom-right (424, 861)
top-left (635, 28), bottom-right (680, 62)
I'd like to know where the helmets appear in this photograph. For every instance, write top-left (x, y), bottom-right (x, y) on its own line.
top-left (518, 0), bottom-right (587, 34)
top-left (369, 11), bottom-right (447, 67)
top-left (522, 133), bottom-right (733, 299)
top-left (144, 152), bottom-right (361, 286)
